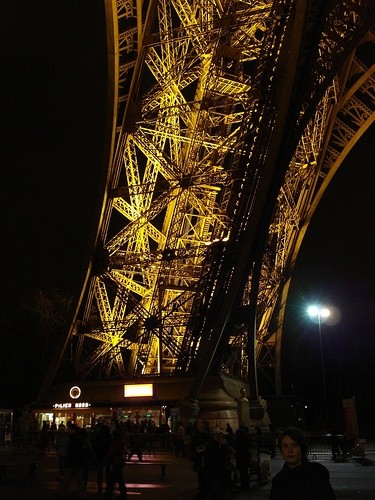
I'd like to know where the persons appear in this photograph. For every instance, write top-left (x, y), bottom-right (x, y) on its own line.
top-left (329, 416), bottom-right (350, 460)
top-left (270, 421), bottom-right (336, 500)
top-left (0, 414), bottom-right (263, 500)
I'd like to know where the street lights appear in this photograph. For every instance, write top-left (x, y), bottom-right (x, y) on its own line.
top-left (308, 304), bottom-right (331, 427)
